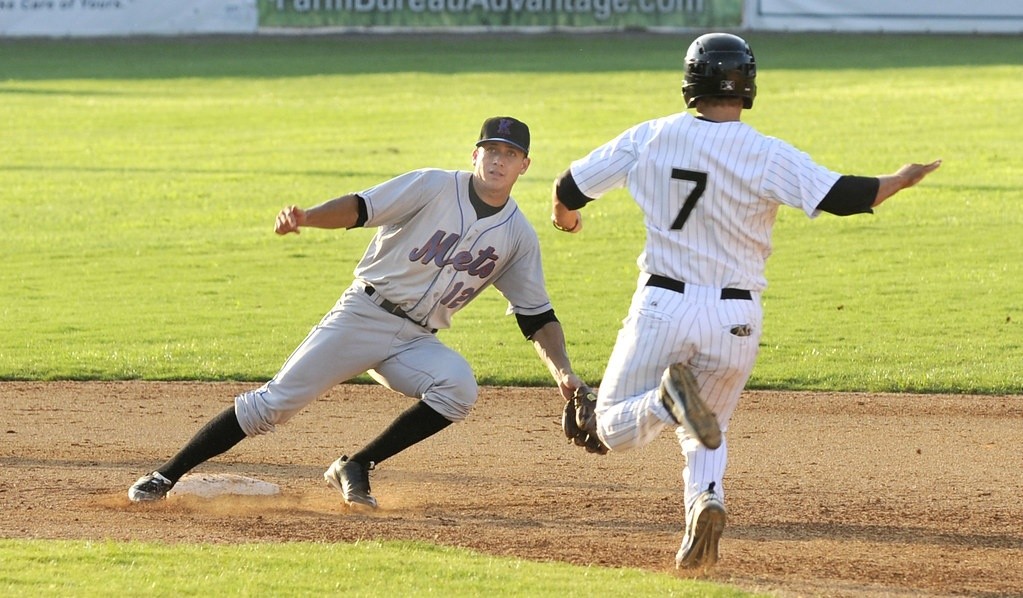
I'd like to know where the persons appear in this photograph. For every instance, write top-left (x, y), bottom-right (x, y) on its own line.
top-left (553, 32), bottom-right (943, 570)
top-left (129, 117), bottom-right (609, 511)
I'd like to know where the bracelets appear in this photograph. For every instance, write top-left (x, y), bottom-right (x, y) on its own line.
top-left (551, 213), bottom-right (578, 232)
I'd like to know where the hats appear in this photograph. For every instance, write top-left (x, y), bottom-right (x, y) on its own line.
top-left (476, 116), bottom-right (530, 154)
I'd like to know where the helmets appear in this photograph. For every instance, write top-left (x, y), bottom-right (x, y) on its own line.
top-left (682, 32), bottom-right (757, 109)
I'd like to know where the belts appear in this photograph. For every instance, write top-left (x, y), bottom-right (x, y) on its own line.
top-left (365, 286), bottom-right (437, 333)
top-left (645, 274), bottom-right (752, 300)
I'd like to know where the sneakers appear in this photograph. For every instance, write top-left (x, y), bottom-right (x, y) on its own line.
top-left (660, 362), bottom-right (721, 449)
top-left (128, 471), bottom-right (172, 502)
top-left (324, 454), bottom-right (377, 510)
top-left (676, 481), bottom-right (726, 569)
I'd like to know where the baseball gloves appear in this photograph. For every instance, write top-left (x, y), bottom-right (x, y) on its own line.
top-left (560, 386), bottom-right (609, 457)
